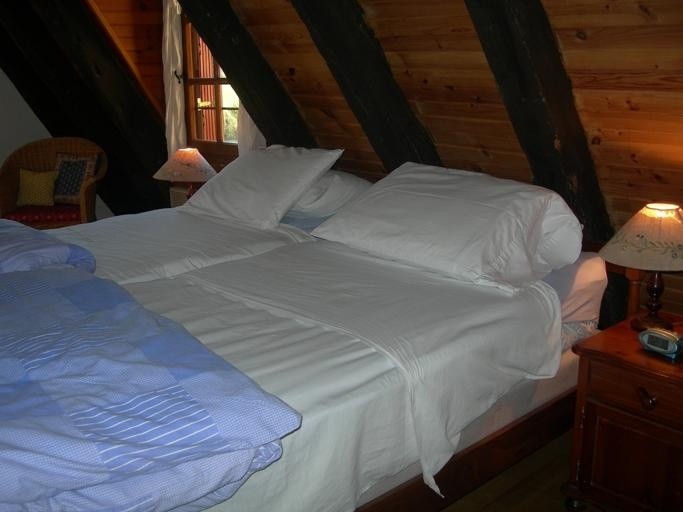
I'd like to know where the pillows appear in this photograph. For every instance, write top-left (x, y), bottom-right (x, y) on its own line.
top-left (183, 146), bottom-right (342, 231)
top-left (311, 161), bottom-right (583, 295)
top-left (295, 170), bottom-right (367, 218)
top-left (15, 163), bottom-right (53, 203)
top-left (54, 150), bottom-right (96, 204)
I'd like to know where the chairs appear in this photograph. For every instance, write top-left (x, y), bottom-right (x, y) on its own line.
top-left (1, 137), bottom-right (102, 230)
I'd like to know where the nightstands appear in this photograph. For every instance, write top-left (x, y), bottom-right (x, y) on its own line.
top-left (572, 310), bottom-right (683, 512)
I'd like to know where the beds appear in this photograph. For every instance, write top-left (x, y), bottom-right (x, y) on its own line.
top-left (0, 205), bottom-right (336, 298)
top-left (0, 238), bottom-right (608, 512)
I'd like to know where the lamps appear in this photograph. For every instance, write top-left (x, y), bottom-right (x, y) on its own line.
top-left (153, 148), bottom-right (216, 182)
top-left (599, 203), bottom-right (683, 331)
